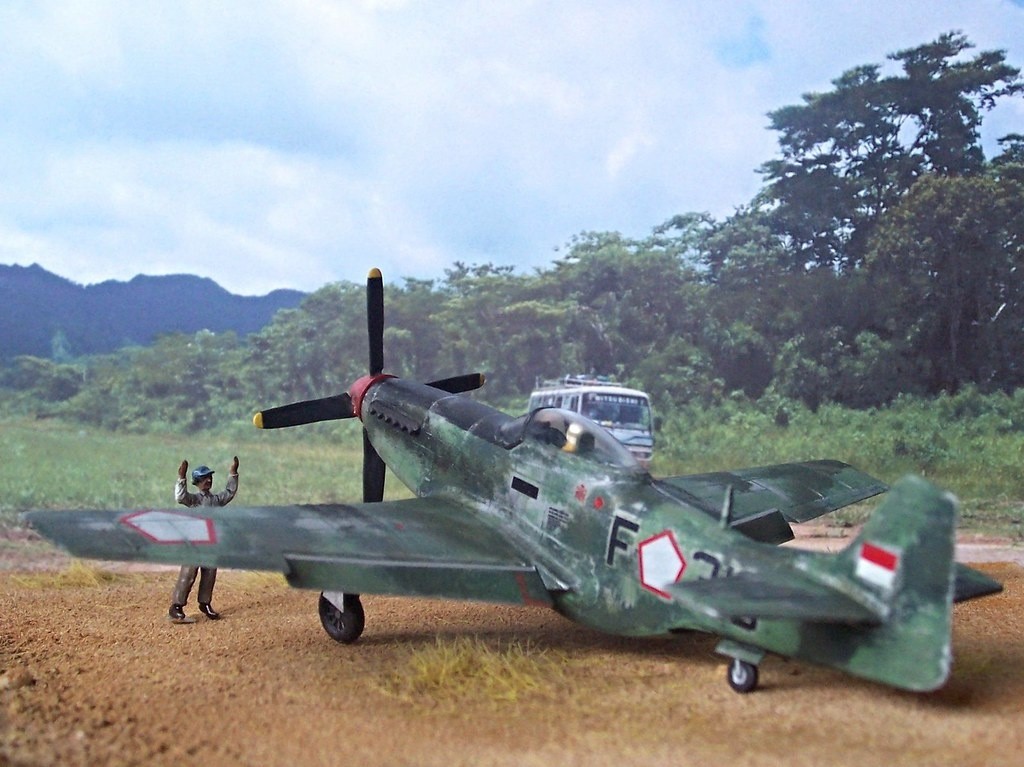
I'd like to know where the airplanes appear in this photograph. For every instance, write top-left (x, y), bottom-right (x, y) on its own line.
top-left (21, 266), bottom-right (1007, 697)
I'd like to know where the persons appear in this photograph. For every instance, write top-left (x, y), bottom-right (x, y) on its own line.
top-left (168, 457), bottom-right (240, 619)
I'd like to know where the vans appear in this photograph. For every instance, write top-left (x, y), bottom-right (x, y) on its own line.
top-left (528, 373), bottom-right (664, 471)
